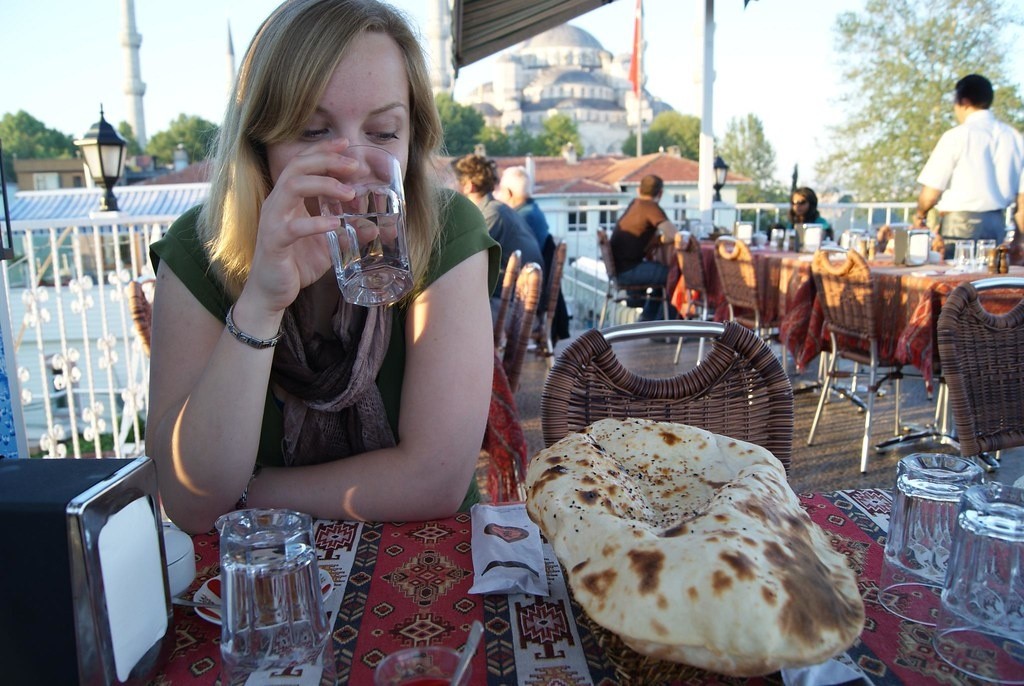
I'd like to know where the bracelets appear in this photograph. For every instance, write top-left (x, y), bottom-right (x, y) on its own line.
top-left (915, 213), bottom-right (926, 220)
top-left (226, 305), bottom-right (286, 349)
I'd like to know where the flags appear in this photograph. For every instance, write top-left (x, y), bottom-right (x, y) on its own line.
top-left (629, 0), bottom-right (642, 97)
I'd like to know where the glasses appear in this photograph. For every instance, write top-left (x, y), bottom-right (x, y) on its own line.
top-left (792, 200), bottom-right (808, 205)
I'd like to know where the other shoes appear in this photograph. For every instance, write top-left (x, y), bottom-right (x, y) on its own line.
top-left (651, 334), bottom-right (687, 343)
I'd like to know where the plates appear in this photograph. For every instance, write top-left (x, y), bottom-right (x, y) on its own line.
top-left (193, 568), bottom-right (334, 626)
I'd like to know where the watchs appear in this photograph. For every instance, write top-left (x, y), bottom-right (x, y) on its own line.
top-left (237, 463), bottom-right (261, 509)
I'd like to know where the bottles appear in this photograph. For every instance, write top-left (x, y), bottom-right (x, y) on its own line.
top-left (842, 229), bottom-right (877, 264)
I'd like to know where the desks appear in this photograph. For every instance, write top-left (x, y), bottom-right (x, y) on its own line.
top-left (666, 239), bottom-right (1023, 473)
top-left (134, 477), bottom-right (1024, 686)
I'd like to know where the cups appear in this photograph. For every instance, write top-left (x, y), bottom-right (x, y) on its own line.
top-left (879, 454), bottom-right (983, 626)
top-left (754, 227), bottom-right (797, 252)
top-left (932, 483), bottom-right (1024, 685)
top-left (374, 647), bottom-right (471, 686)
top-left (214, 508), bottom-right (338, 686)
top-left (953, 238), bottom-right (995, 274)
top-left (318, 146), bottom-right (413, 307)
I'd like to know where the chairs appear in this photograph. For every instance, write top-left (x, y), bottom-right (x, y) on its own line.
top-left (493, 227), bottom-right (1024, 465)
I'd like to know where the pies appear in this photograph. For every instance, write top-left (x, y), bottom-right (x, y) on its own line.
top-left (521, 414), bottom-right (863, 678)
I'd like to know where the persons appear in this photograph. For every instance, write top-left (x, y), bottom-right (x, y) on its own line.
top-left (789, 187), bottom-right (834, 239)
top-left (455, 154), bottom-right (545, 332)
top-left (499, 165), bottom-right (569, 339)
top-left (912, 74), bottom-right (1024, 264)
top-left (144, 0), bottom-right (502, 533)
top-left (610, 175), bottom-right (678, 322)
